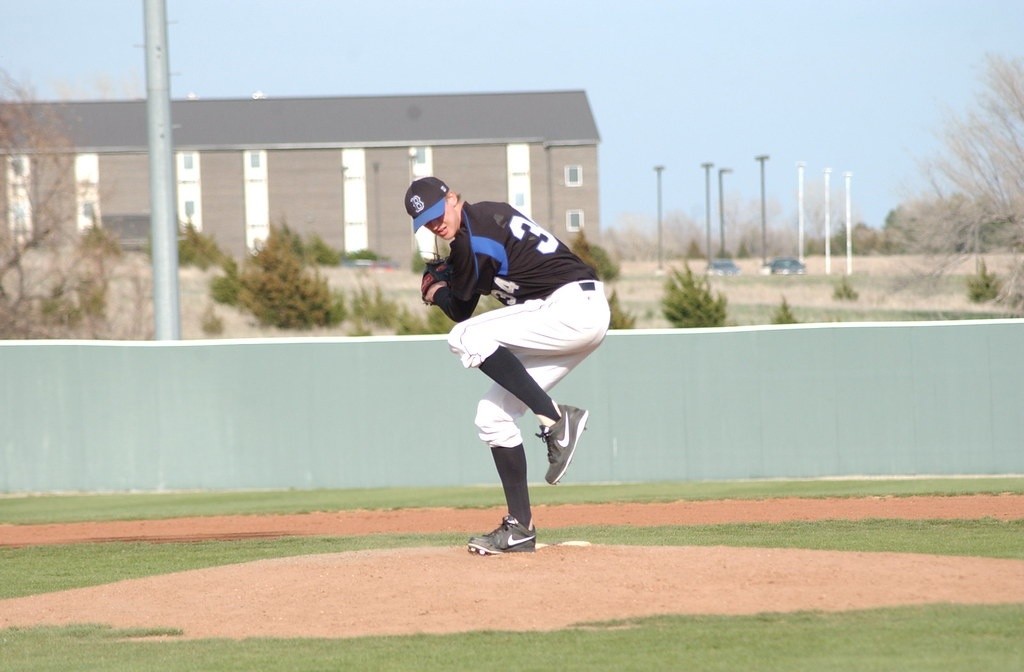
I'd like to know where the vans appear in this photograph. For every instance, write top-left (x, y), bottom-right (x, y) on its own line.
top-left (100, 210), bottom-right (188, 265)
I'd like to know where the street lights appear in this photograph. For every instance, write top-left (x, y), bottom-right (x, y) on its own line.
top-left (701, 162), bottom-right (714, 259)
top-left (719, 169), bottom-right (732, 250)
top-left (756, 156), bottom-right (769, 266)
top-left (654, 166), bottom-right (666, 270)
top-left (843, 171), bottom-right (853, 275)
top-left (822, 167), bottom-right (833, 275)
top-left (796, 160), bottom-right (807, 265)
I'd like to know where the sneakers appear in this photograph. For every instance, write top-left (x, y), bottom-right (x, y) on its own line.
top-left (536, 405), bottom-right (589, 485)
top-left (467, 514), bottom-right (536, 555)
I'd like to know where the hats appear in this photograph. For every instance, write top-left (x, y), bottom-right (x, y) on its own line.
top-left (405, 177), bottom-right (450, 233)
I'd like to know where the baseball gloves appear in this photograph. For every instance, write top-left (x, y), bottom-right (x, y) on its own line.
top-left (422, 257), bottom-right (450, 306)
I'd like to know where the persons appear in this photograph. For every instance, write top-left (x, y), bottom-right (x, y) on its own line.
top-left (405, 177), bottom-right (612, 556)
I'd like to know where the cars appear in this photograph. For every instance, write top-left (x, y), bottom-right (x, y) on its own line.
top-left (704, 262), bottom-right (741, 278)
top-left (770, 258), bottom-right (807, 276)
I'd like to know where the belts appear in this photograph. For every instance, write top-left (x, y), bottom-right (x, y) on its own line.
top-left (578, 282), bottom-right (595, 291)
